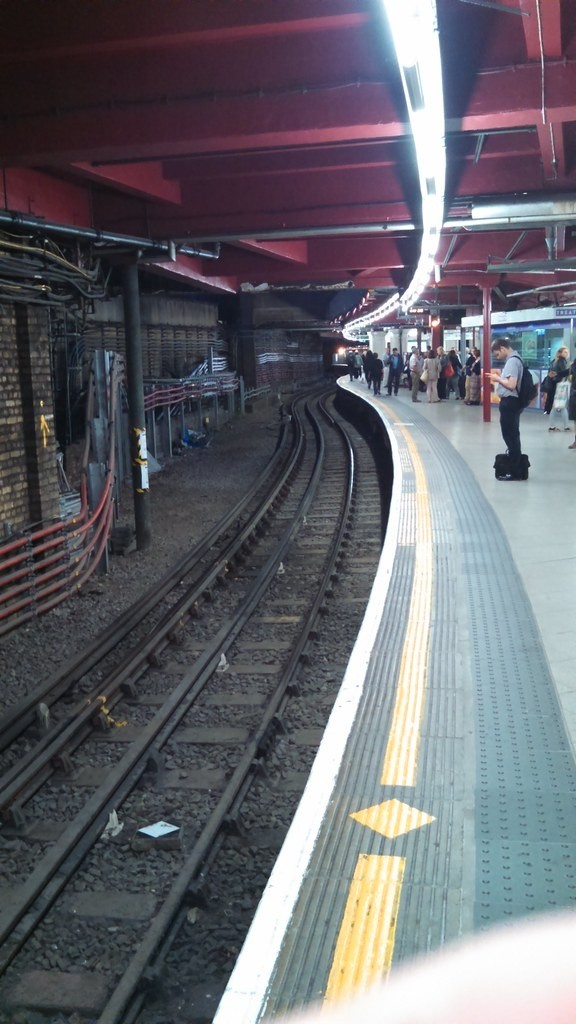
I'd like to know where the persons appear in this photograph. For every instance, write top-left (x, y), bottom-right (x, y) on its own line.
top-left (548, 358), bottom-right (576, 449)
top-left (544, 346), bottom-right (571, 432)
top-left (483, 338), bottom-right (524, 455)
top-left (346, 343), bottom-right (480, 405)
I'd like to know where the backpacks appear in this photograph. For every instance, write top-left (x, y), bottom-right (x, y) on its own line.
top-left (509, 354), bottom-right (539, 412)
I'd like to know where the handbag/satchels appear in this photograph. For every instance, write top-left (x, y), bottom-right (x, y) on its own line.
top-left (443, 362), bottom-right (455, 378)
top-left (420, 368), bottom-right (428, 383)
top-left (554, 380), bottom-right (571, 410)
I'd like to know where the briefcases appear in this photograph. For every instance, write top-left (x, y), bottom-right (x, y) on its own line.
top-left (492, 452), bottom-right (532, 481)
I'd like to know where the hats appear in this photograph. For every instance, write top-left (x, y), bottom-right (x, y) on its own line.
top-left (411, 345), bottom-right (417, 352)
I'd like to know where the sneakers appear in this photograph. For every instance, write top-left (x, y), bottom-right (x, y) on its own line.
top-left (548, 425), bottom-right (557, 432)
top-left (563, 425), bottom-right (571, 432)
